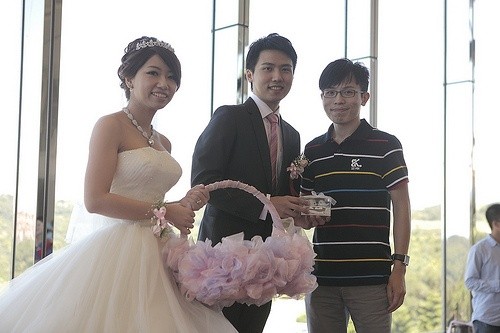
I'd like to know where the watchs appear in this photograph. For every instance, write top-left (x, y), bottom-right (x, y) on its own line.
top-left (391, 253), bottom-right (410, 265)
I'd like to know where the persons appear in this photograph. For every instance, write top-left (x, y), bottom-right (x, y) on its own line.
top-left (464, 202), bottom-right (500, 333)
top-left (0, 35), bottom-right (237, 333)
top-left (300, 58), bottom-right (411, 333)
top-left (191, 33), bottom-right (330, 333)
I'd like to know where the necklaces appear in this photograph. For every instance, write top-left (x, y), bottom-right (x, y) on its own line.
top-left (122, 107), bottom-right (155, 147)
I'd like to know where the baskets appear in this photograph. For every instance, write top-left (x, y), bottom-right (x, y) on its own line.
top-left (163, 180), bottom-right (318, 310)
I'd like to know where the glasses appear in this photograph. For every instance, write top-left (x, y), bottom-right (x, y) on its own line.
top-left (322, 88), bottom-right (365, 98)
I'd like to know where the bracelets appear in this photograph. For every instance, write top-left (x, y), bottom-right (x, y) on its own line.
top-left (152, 199), bottom-right (174, 239)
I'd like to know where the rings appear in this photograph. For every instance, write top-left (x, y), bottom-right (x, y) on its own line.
top-left (197, 200), bottom-right (201, 203)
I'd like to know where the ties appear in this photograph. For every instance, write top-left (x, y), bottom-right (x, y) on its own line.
top-left (265, 114), bottom-right (279, 183)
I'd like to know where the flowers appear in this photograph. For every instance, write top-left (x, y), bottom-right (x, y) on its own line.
top-left (287, 152), bottom-right (310, 177)
